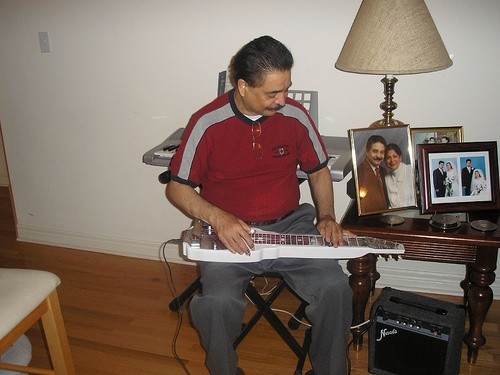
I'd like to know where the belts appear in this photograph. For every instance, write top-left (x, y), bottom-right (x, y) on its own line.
top-left (245, 209), bottom-right (296, 226)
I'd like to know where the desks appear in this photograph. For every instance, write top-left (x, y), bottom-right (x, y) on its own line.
top-left (340, 203), bottom-right (500, 366)
top-left (145, 129), bottom-right (351, 326)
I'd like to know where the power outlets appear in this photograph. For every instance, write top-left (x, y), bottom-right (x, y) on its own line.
top-left (38, 31), bottom-right (51, 54)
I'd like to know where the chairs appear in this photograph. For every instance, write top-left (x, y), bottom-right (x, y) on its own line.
top-left (0, 268), bottom-right (76, 375)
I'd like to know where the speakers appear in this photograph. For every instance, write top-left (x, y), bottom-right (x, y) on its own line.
top-left (366, 285), bottom-right (467, 375)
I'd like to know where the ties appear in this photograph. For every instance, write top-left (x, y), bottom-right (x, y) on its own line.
top-left (375, 168), bottom-right (383, 185)
top-left (442, 170), bottom-right (445, 175)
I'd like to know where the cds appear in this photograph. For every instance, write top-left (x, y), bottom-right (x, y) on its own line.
top-left (432, 215), bottom-right (458, 227)
top-left (381, 214), bottom-right (404, 224)
top-left (471, 219), bottom-right (498, 232)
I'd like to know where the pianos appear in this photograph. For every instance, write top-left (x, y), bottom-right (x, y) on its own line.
top-left (142, 127), bottom-right (353, 330)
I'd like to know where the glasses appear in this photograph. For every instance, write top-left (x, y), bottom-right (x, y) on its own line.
top-left (251, 121), bottom-right (263, 160)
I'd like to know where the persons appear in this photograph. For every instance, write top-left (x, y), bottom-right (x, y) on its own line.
top-left (385, 143), bottom-right (415, 207)
top-left (442, 137), bottom-right (449, 143)
top-left (461, 159), bottom-right (487, 195)
top-left (168, 36), bottom-right (354, 375)
top-left (433, 161), bottom-right (460, 197)
top-left (429, 137), bottom-right (435, 144)
top-left (358, 135), bottom-right (390, 213)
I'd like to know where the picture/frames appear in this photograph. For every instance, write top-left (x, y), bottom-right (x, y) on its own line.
top-left (409, 126), bottom-right (465, 209)
top-left (417, 141), bottom-right (500, 215)
top-left (348, 123), bottom-right (418, 217)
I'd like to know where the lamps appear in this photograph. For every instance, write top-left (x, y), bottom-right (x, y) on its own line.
top-left (334, 0), bottom-right (453, 128)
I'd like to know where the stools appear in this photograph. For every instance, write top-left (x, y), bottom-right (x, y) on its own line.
top-left (232, 273), bottom-right (312, 375)
